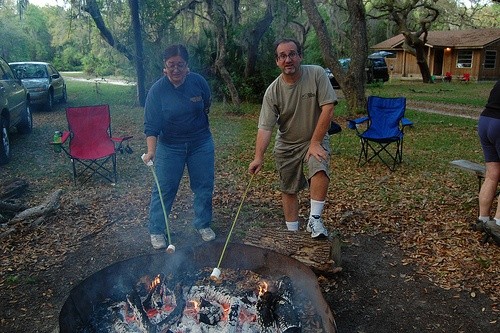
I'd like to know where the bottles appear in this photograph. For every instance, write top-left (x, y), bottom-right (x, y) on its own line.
top-left (53, 130), bottom-right (62, 153)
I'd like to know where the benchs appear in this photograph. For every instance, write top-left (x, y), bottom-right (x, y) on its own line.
top-left (447, 159), bottom-right (500, 217)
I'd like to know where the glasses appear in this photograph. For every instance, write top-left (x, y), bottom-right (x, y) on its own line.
top-left (165, 62), bottom-right (187, 69)
top-left (276, 52), bottom-right (300, 60)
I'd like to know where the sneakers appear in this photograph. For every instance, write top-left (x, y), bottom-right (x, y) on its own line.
top-left (195, 226), bottom-right (216, 241)
top-left (307, 215), bottom-right (328, 239)
top-left (150, 234), bottom-right (167, 249)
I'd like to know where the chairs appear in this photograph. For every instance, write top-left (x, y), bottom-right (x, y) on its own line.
top-left (347, 95), bottom-right (414, 172)
top-left (49, 104), bottom-right (134, 190)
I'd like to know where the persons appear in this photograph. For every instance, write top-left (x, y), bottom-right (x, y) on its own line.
top-left (248, 38), bottom-right (339, 238)
top-left (142, 44), bottom-right (216, 249)
top-left (476, 70), bottom-right (500, 247)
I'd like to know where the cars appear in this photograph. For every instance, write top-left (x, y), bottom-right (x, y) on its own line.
top-left (9, 61), bottom-right (67, 111)
top-left (0, 56), bottom-right (33, 165)
top-left (324, 50), bottom-right (394, 88)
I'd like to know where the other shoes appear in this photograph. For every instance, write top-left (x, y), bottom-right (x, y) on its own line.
top-left (475, 219), bottom-right (486, 231)
top-left (483, 220), bottom-right (500, 244)
